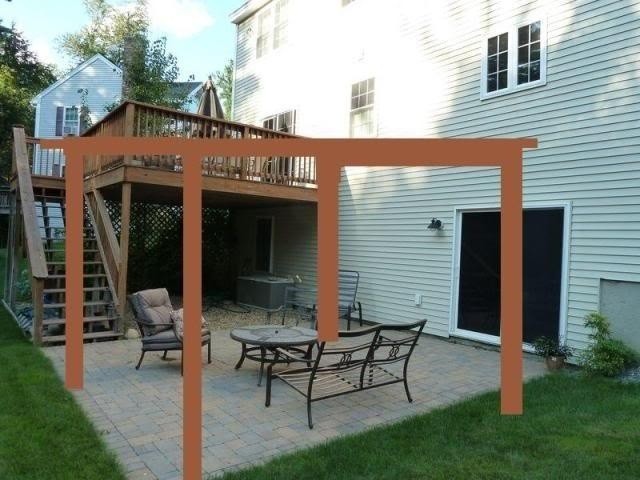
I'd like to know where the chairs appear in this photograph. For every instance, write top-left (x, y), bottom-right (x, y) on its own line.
top-left (311, 269), bottom-right (363, 331)
top-left (126, 288), bottom-right (212, 376)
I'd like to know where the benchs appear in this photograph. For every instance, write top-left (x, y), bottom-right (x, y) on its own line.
top-left (265, 318), bottom-right (428, 429)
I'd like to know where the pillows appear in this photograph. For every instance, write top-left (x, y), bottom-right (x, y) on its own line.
top-left (169, 307), bottom-right (208, 340)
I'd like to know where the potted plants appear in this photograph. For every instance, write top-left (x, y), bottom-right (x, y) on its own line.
top-left (104, 34), bottom-right (193, 169)
top-left (530, 335), bottom-right (576, 371)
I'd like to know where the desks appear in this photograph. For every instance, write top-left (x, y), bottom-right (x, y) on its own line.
top-left (229, 324), bottom-right (319, 386)
top-left (235, 274), bottom-right (294, 312)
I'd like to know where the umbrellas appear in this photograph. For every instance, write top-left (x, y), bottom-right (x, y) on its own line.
top-left (190, 72), bottom-right (231, 176)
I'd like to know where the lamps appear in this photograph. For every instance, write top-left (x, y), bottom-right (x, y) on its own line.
top-left (427, 217), bottom-right (441, 233)
